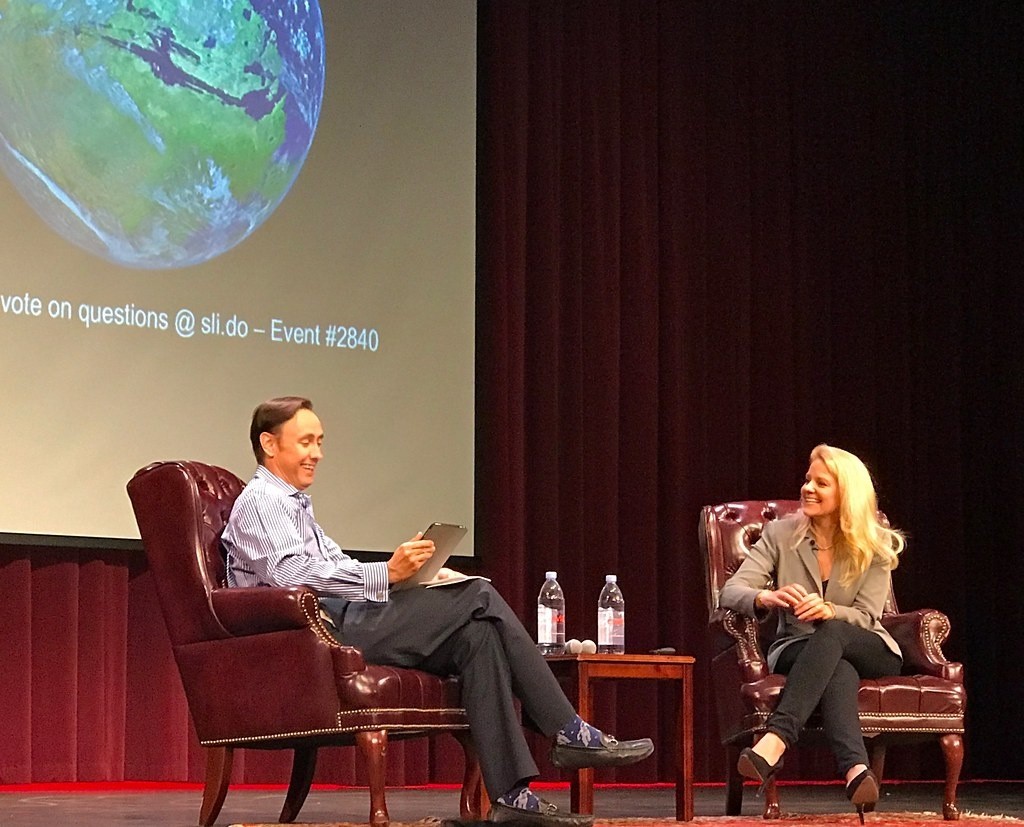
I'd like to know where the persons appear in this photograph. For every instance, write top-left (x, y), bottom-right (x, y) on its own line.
top-left (718, 444), bottom-right (908, 826)
top-left (219, 396), bottom-right (655, 827)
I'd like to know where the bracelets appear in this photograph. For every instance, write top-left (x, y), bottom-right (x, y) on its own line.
top-left (825, 601), bottom-right (835, 621)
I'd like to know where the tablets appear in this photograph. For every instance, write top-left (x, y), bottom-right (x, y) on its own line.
top-left (391, 522), bottom-right (469, 596)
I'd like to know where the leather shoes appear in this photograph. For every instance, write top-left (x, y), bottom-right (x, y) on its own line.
top-left (549, 732), bottom-right (655, 769)
top-left (487, 798), bottom-right (595, 827)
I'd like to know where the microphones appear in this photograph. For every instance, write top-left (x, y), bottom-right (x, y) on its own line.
top-left (811, 540), bottom-right (815, 545)
top-left (564, 640), bottom-right (596, 657)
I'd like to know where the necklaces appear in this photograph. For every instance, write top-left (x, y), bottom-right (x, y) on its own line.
top-left (817, 544), bottom-right (832, 550)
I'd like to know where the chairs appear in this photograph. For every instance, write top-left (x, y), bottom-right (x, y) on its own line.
top-left (697, 499), bottom-right (966, 822)
top-left (124, 459), bottom-right (480, 827)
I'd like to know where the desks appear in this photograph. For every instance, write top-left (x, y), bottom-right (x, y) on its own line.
top-left (481, 654), bottom-right (695, 822)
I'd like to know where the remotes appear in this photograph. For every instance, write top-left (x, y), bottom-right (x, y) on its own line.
top-left (648, 648), bottom-right (676, 655)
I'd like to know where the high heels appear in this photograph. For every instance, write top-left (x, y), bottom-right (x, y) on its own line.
top-left (737, 747), bottom-right (783, 797)
top-left (846, 769), bottom-right (878, 825)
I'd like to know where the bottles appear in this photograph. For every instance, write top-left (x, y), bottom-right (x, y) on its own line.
top-left (596, 573), bottom-right (626, 655)
top-left (535, 571), bottom-right (566, 656)
